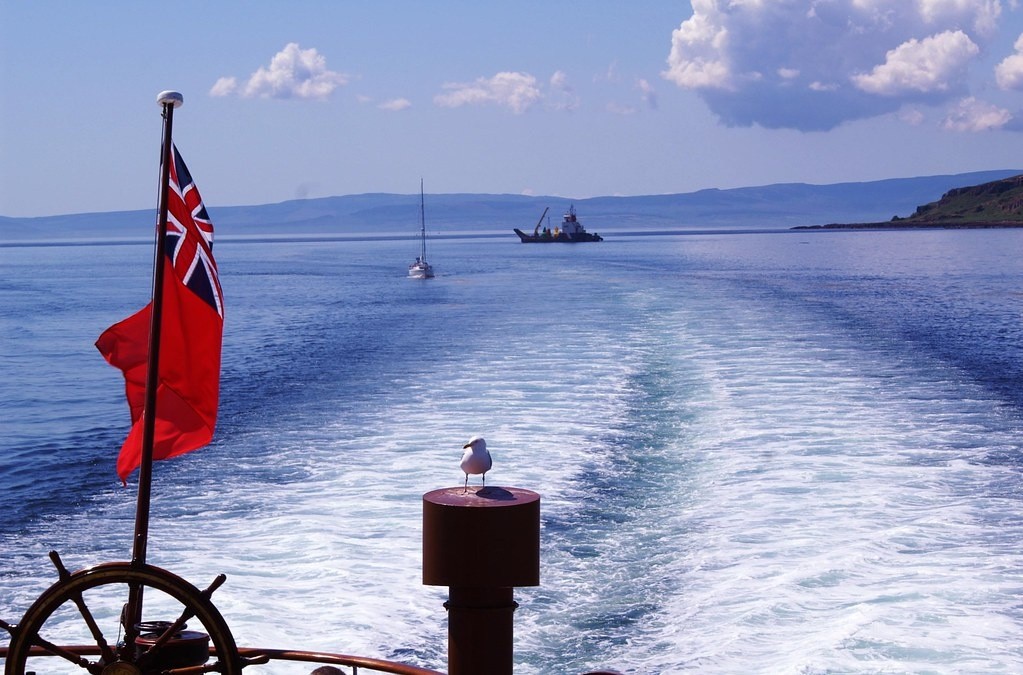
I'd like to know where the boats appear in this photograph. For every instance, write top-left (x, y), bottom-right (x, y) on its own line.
top-left (513, 203), bottom-right (605, 245)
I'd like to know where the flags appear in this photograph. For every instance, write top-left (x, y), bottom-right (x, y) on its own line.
top-left (94, 136), bottom-right (226, 487)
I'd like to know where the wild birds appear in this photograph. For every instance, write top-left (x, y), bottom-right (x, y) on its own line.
top-left (460, 436), bottom-right (493, 492)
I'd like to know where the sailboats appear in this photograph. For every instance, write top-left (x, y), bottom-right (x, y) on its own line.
top-left (405, 177), bottom-right (438, 279)
